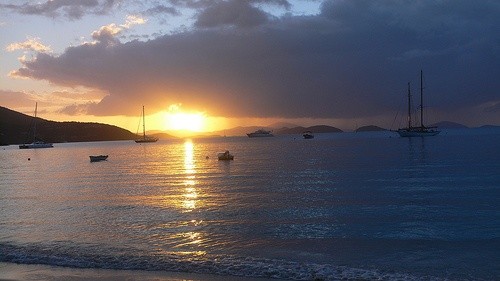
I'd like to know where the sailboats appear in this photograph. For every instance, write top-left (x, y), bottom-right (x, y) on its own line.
top-left (134, 104), bottom-right (159, 143)
top-left (19, 102), bottom-right (54, 148)
top-left (396, 67), bottom-right (440, 137)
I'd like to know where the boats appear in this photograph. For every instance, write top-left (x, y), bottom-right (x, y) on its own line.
top-left (89, 154), bottom-right (108, 162)
top-left (247, 129), bottom-right (273, 137)
top-left (218, 150), bottom-right (234, 160)
top-left (303, 134), bottom-right (314, 139)
